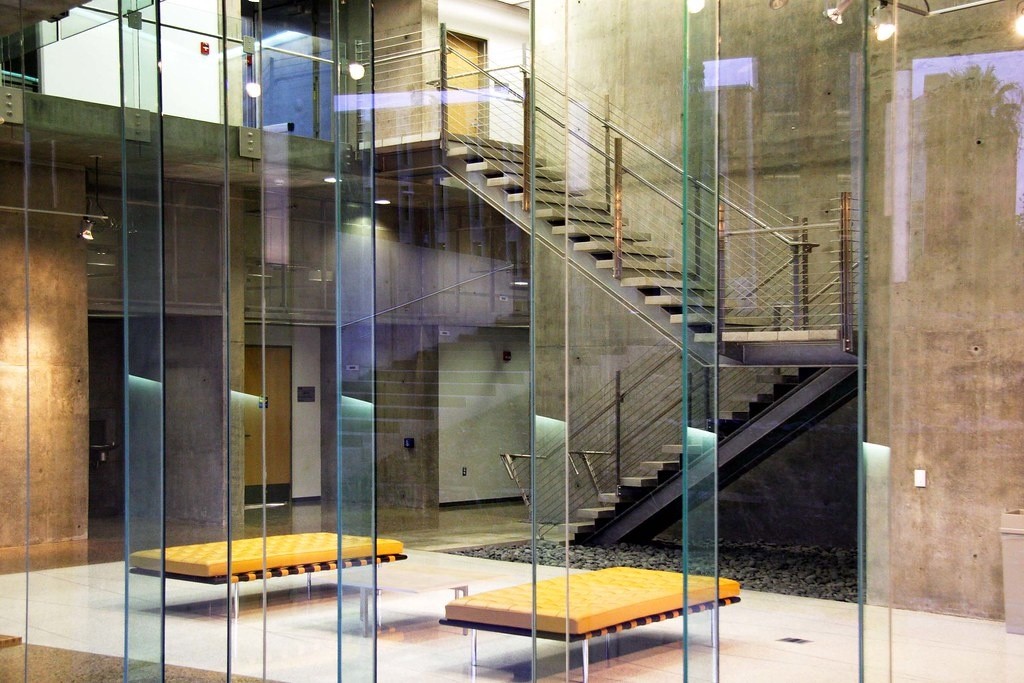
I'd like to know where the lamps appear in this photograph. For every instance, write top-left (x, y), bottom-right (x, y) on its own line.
top-left (770, 0), bottom-right (788, 11)
top-left (822, 0), bottom-right (853, 25)
top-left (869, 15), bottom-right (895, 42)
top-left (82, 224), bottom-right (94, 240)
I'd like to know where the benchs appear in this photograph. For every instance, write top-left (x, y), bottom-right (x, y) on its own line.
top-left (129, 533), bottom-right (408, 625)
top-left (438, 567), bottom-right (740, 682)
